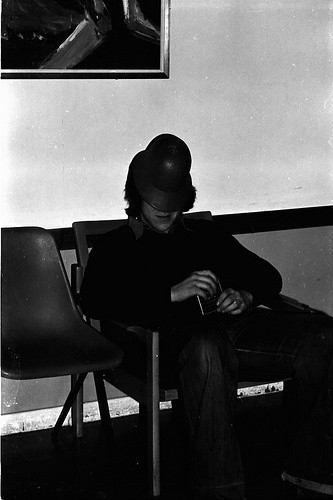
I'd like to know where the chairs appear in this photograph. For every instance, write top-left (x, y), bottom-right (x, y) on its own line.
top-left (1, 227), bottom-right (124, 470)
top-left (71, 211), bottom-right (317, 497)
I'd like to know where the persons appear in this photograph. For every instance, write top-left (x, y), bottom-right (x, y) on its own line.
top-left (78, 133), bottom-right (333, 500)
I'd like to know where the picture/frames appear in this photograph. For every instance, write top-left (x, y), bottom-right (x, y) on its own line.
top-left (1, 0), bottom-right (170, 79)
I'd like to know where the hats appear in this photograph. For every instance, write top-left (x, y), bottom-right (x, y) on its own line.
top-left (130, 134), bottom-right (192, 212)
